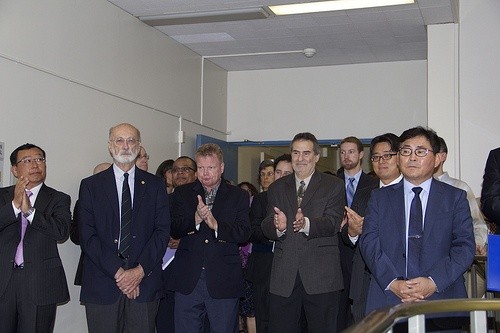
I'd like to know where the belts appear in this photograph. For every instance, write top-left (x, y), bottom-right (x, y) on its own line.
top-left (13, 263), bottom-right (24, 270)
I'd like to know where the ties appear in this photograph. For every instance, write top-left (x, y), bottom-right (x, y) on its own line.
top-left (15, 190), bottom-right (34, 266)
top-left (407, 186), bottom-right (423, 280)
top-left (119, 173), bottom-right (132, 270)
top-left (296, 181), bottom-right (306, 207)
top-left (345, 177), bottom-right (356, 210)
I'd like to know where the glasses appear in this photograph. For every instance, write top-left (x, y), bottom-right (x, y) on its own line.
top-left (16, 156), bottom-right (46, 167)
top-left (171, 166), bottom-right (196, 176)
top-left (260, 170), bottom-right (274, 178)
top-left (136, 155), bottom-right (149, 160)
top-left (370, 153), bottom-right (397, 163)
top-left (398, 146), bottom-right (433, 157)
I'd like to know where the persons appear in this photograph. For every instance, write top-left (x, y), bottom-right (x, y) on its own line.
top-left (71, 122), bottom-right (500, 333)
top-left (0, 143), bottom-right (73, 333)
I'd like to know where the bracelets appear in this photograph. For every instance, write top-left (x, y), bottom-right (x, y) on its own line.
top-left (23, 207), bottom-right (34, 218)
